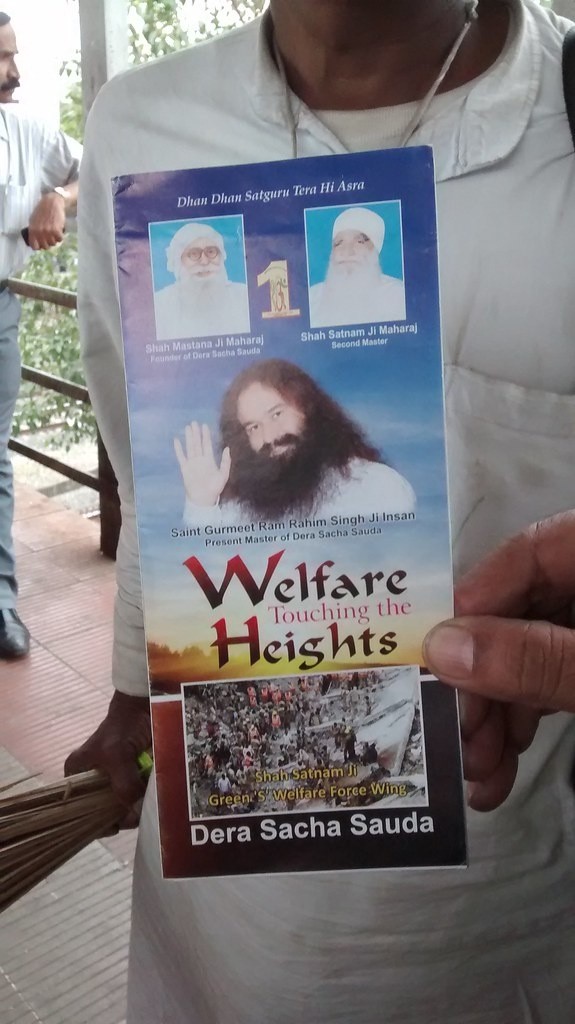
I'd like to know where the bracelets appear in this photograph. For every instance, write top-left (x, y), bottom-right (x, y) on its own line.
top-left (50, 186), bottom-right (70, 207)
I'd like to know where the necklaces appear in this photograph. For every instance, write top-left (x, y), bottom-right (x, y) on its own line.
top-left (268, 0), bottom-right (479, 159)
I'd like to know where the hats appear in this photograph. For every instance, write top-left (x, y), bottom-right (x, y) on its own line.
top-left (332, 207), bottom-right (386, 254)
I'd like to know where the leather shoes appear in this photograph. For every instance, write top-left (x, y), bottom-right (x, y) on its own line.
top-left (1, 607), bottom-right (32, 659)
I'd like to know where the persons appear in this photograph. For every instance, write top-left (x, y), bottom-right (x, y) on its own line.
top-left (182, 666), bottom-right (400, 813)
top-left (309, 207), bottom-right (406, 328)
top-left (63, 0), bottom-right (573, 1024)
top-left (1, 11), bottom-right (85, 654)
top-left (420, 506), bottom-right (574, 811)
top-left (154, 222), bottom-right (251, 341)
top-left (173, 360), bottom-right (416, 527)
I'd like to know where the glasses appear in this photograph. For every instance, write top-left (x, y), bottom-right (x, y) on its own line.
top-left (183, 247), bottom-right (223, 262)
